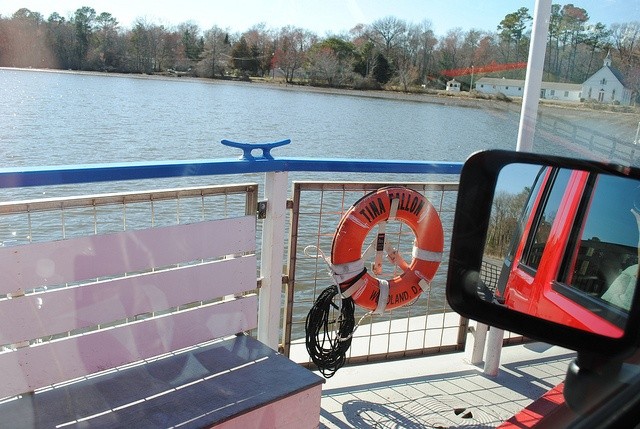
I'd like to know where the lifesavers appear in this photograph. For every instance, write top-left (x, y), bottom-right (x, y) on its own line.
top-left (330, 187), bottom-right (444, 311)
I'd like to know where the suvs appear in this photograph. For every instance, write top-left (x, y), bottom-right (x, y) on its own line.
top-left (493, 165), bottom-right (639, 338)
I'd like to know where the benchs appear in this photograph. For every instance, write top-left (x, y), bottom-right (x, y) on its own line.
top-left (0, 216), bottom-right (326, 429)
